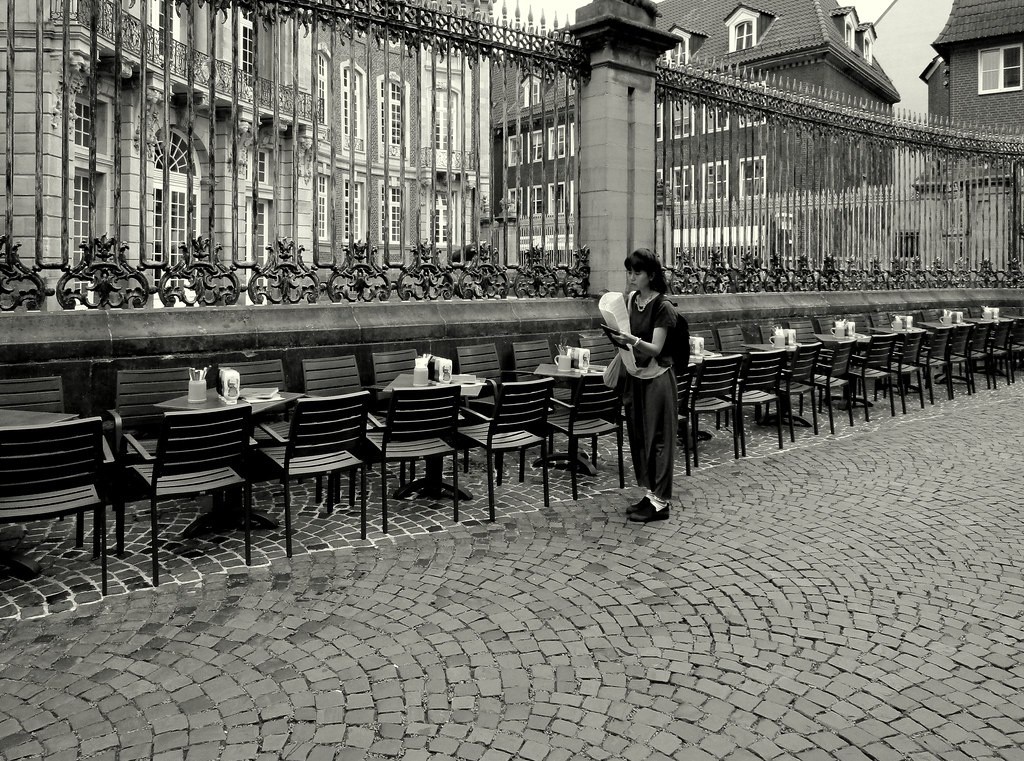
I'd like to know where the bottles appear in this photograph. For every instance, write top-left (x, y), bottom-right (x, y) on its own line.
top-left (429, 356), bottom-right (453, 386)
top-left (566, 347), bottom-right (590, 374)
top-left (216, 367), bottom-right (241, 405)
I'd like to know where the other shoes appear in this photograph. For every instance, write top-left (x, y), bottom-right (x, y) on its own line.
top-left (630, 500), bottom-right (669, 522)
top-left (626, 496), bottom-right (650, 514)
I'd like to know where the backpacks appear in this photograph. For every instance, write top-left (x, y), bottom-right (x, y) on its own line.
top-left (631, 290), bottom-right (690, 376)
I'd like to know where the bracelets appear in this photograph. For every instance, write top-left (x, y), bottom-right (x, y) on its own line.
top-left (632, 337), bottom-right (642, 347)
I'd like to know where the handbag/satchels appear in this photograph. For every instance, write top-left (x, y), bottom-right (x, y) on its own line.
top-left (599, 289), bottom-right (637, 390)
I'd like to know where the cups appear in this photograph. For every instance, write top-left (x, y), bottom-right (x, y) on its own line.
top-left (690, 337), bottom-right (704, 356)
top-left (940, 316), bottom-right (952, 326)
top-left (554, 354), bottom-right (571, 372)
top-left (413, 367), bottom-right (428, 386)
top-left (831, 327), bottom-right (844, 337)
top-left (769, 335), bottom-right (786, 349)
top-left (188, 381), bottom-right (207, 403)
top-left (982, 311), bottom-right (992, 321)
top-left (891, 320), bottom-right (903, 331)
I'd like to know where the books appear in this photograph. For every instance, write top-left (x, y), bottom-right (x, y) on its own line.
top-left (855, 334), bottom-right (866, 338)
top-left (451, 373), bottom-right (487, 387)
top-left (785, 343), bottom-right (802, 348)
top-left (588, 365), bottom-right (608, 374)
top-left (908, 327), bottom-right (926, 332)
top-left (701, 350), bottom-right (722, 358)
top-left (238, 387), bottom-right (285, 404)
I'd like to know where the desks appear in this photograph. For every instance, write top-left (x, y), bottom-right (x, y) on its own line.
top-left (1004, 312), bottom-right (1024, 322)
top-left (382, 372), bottom-right (489, 501)
top-left (919, 320), bottom-right (974, 385)
top-left (678, 348), bottom-right (723, 441)
top-left (812, 330), bottom-right (874, 408)
top-left (961, 315), bottom-right (1013, 378)
top-left (153, 384), bottom-right (305, 535)
top-left (739, 341), bottom-right (814, 427)
top-left (871, 324), bottom-right (928, 395)
top-left (0, 408), bottom-right (81, 575)
top-left (532, 363), bottom-right (608, 474)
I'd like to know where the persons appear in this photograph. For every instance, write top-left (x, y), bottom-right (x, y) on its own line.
top-left (611, 248), bottom-right (677, 522)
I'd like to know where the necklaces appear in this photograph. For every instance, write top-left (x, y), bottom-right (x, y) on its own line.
top-left (637, 294), bottom-right (652, 311)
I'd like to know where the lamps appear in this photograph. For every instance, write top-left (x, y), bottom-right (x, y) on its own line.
top-left (943, 69), bottom-right (949, 74)
top-left (943, 80), bottom-right (949, 87)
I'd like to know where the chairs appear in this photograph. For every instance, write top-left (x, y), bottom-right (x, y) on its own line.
top-left (0, 306), bottom-right (1024, 596)
